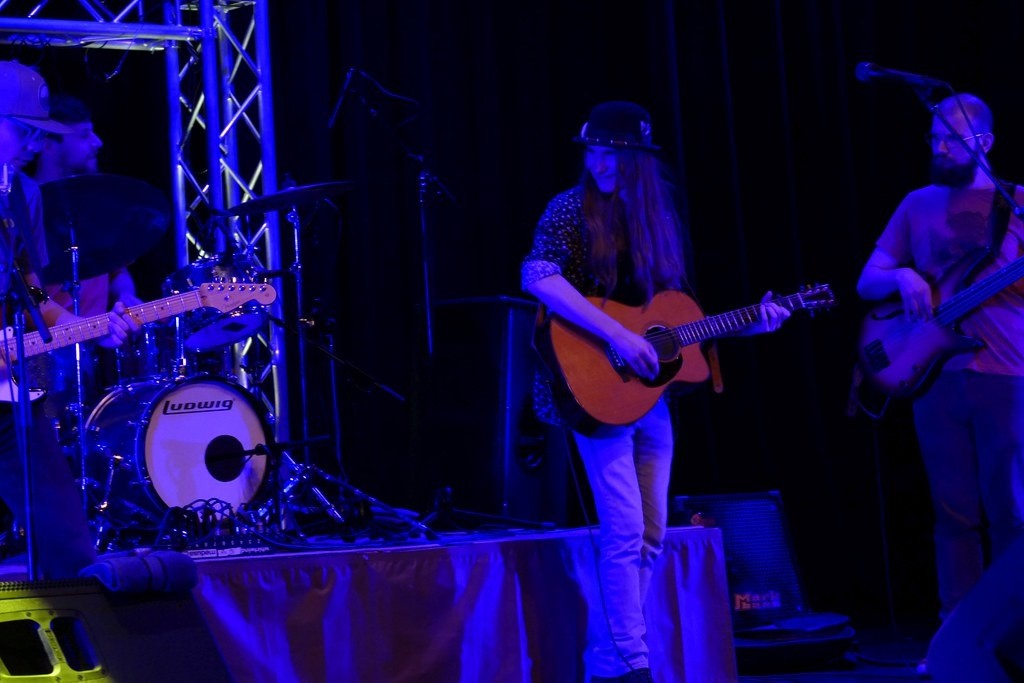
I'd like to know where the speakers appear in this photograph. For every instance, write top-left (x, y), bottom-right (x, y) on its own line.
top-left (0, 580), bottom-right (236, 683)
top-left (673, 492), bottom-right (806, 632)
top-left (416, 294), bottom-right (568, 529)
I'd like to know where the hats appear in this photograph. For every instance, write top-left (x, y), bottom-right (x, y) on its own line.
top-left (573, 102), bottom-right (662, 154)
top-left (0, 60), bottom-right (73, 136)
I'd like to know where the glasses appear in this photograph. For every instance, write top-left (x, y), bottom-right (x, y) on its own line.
top-left (4, 118), bottom-right (49, 141)
top-left (926, 131), bottom-right (986, 150)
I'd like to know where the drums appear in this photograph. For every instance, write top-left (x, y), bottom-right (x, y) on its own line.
top-left (74, 374), bottom-right (277, 531)
top-left (159, 254), bottom-right (274, 354)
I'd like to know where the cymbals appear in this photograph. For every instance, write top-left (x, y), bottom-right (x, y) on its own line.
top-left (222, 181), bottom-right (356, 216)
top-left (39, 174), bottom-right (173, 283)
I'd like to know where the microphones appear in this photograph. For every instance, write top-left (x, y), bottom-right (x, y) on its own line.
top-left (856, 63), bottom-right (941, 87)
top-left (328, 68), bottom-right (357, 129)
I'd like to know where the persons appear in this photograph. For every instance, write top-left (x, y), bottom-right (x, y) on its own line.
top-left (0, 58), bottom-right (149, 578)
top-left (858, 96), bottom-right (1024, 619)
top-left (521, 103), bottom-right (791, 683)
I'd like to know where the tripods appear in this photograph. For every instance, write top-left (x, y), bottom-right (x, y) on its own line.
top-left (247, 203), bottom-right (442, 547)
top-left (349, 86), bottom-right (561, 542)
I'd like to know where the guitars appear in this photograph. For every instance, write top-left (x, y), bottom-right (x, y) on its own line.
top-left (541, 282), bottom-right (836, 437)
top-left (857, 253), bottom-right (1023, 405)
top-left (0, 277), bottom-right (275, 406)
